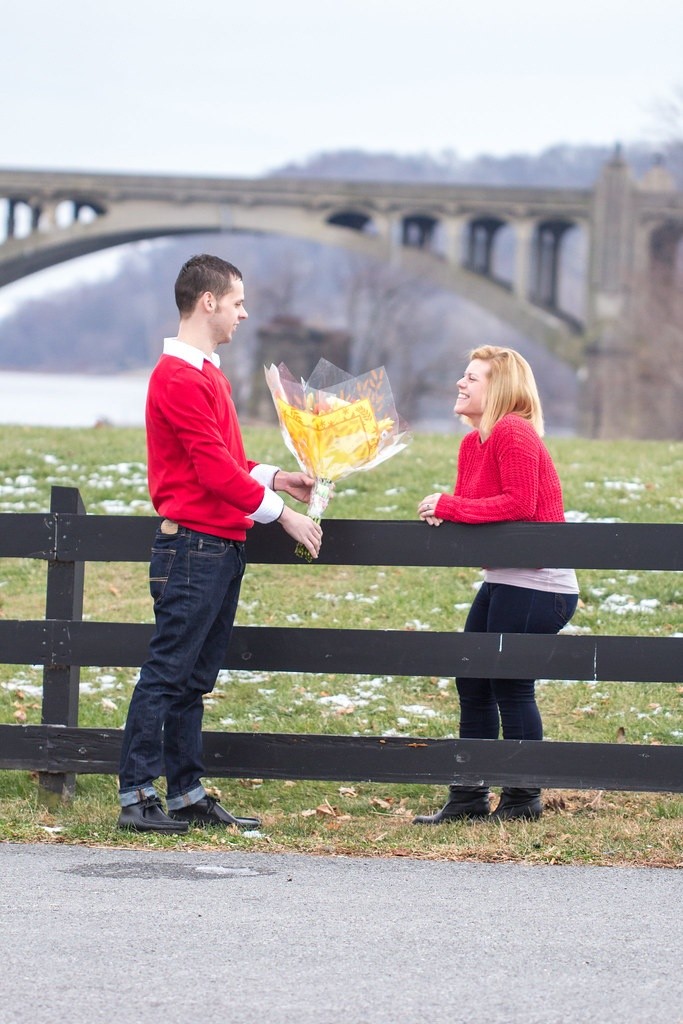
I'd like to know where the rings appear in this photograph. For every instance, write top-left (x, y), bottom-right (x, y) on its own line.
top-left (426, 504), bottom-right (430, 508)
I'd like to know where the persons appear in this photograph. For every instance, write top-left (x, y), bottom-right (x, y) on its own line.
top-left (414, 344), bottom-right (580, 823)
top-left (116, 256), bottom-right (324, 837)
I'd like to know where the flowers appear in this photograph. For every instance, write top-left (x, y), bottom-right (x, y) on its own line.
top-left (261, 355), bottom-right (411, 556)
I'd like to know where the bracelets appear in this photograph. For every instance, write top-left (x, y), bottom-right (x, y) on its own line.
top-left (272, 470), bottom-right (279, 492)
top-left (274, 506), bottom-right (284, 521)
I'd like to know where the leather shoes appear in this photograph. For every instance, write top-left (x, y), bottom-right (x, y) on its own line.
top-left (466, 789), bottom-right (542, 827)
top-left (117, 795), bottom-right (189, 835)
top-left (167, 796), bottom-right (263, 832)
top-left (412, 786), bottom-right (490, 826)
